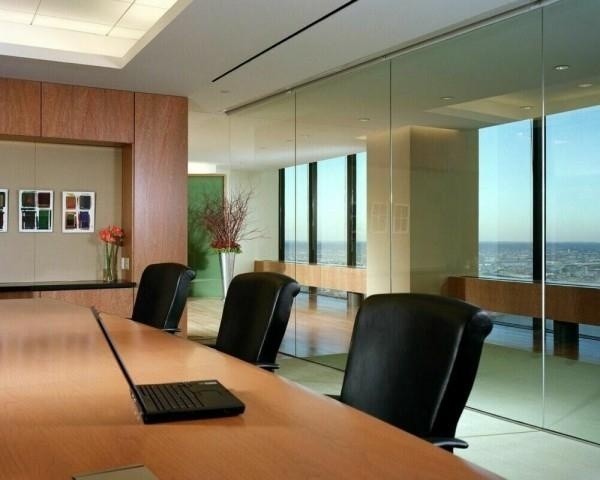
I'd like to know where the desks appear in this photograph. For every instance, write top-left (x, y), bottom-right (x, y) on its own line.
top-left (0, 294), bottom-right (514, 480)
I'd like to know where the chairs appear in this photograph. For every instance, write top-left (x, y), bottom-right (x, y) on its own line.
top-left (204, 271), bottom-right (301, 372)
top-left (323, 292), bottom-right (494, 454)
top-left (126, 262), bottom-right (198, 335)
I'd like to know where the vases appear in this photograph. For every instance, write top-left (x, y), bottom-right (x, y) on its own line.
top-left (219, 248), bottom-right (235, 301)
top-left (102, 241), bottom-right (118, 283)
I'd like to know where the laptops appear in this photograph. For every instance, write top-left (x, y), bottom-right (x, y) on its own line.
top-left (91, 305), bottom-right (246, 425)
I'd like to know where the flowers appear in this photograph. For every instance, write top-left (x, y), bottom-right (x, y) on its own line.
top-left (100, 225), bottom-right (128, 284)
top-left (188, 180), bottom-right (271, 254)
